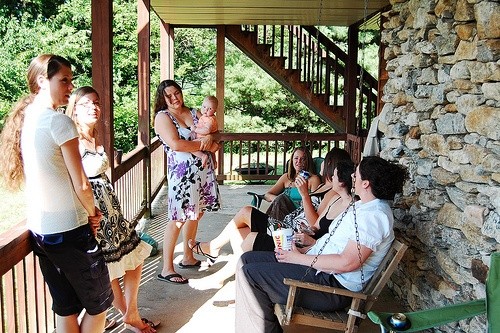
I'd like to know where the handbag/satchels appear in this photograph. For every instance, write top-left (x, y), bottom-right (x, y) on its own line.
top-left (135, 230), bottom-right (158, 256)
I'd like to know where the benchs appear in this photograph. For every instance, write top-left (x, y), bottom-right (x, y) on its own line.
top-left (274, 238), bottom-right (408, 333)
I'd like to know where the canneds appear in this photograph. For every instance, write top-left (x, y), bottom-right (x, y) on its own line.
top-left (389, 312), bottom-right (407, 329)
top-left (298, 170), bottom-right (310, 180)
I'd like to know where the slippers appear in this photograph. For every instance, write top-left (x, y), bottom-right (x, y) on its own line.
top-left (178, 260), bottom-right (211, 269)
top-left (157, 273), bottom-right (190, 284)
top-left (103, 318), bottom-right (115, 330)
top-left (124, 318), bottom-right (161, 329)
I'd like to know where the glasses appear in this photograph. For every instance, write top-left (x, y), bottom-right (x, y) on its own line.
top-left (74, 101), bottom-right (103, 108)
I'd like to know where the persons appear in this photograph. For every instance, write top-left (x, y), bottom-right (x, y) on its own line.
top-left (0, 54), bottom-right (114, 333)
top-left (235, 156), bottom-right (404, 333)
top-left (187, 161), bottom-right (359, 290)
top-left (190, 96), bottom-right (218, 169)
top-left (266, 147), bottom-right (353, 238)
top-left (65, 86), bottom-right (157, 333)
top-left (264, 147), bottom-right (320, 222)
top-left (153, 80), bottom-right (221, 284)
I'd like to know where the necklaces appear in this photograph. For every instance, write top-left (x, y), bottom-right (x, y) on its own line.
top-left (90, 138), bottom-right (98, 155)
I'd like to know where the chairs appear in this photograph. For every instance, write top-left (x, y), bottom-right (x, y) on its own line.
top-left (252, 158), bottom-right (325, 208)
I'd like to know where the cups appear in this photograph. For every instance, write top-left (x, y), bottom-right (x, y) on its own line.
top-left (272, 229), bottom-right (293, 252)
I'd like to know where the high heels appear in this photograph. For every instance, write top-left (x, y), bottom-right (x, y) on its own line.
top-left (187, 239), bottom-right (218, 263)
